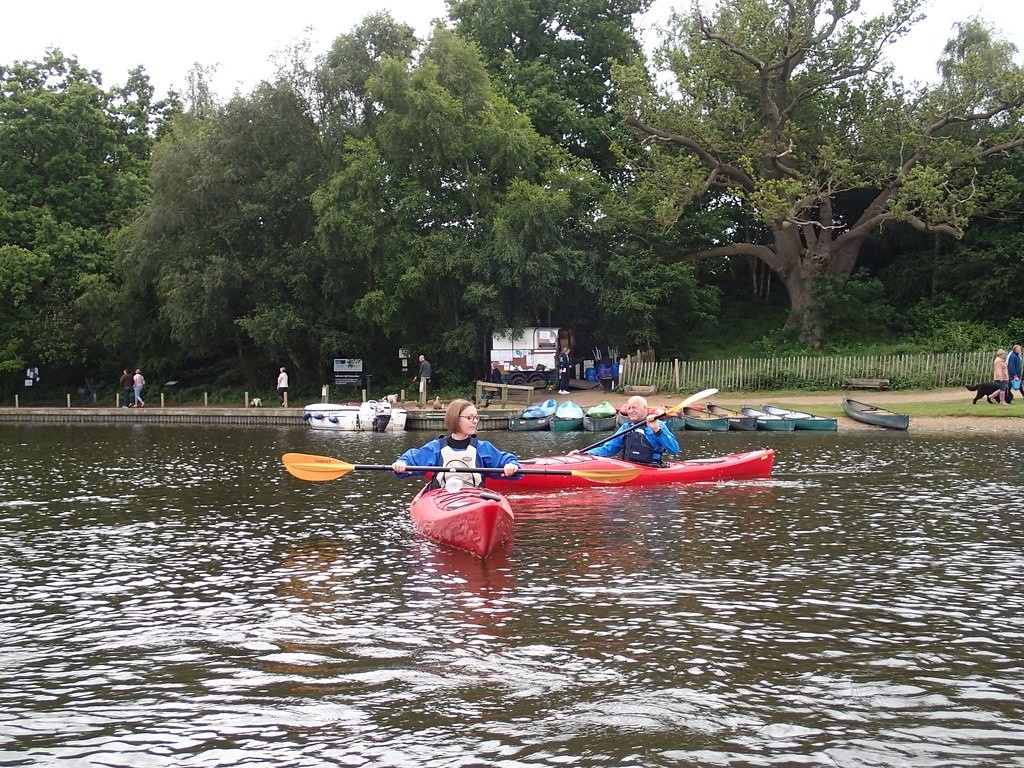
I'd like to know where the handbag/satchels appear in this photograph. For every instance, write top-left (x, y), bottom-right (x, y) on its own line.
top-left (1011, 377), bottom-right (1021, 390)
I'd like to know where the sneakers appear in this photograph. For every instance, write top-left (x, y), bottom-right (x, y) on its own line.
top-left (559, 389), bottom-right (570, 394)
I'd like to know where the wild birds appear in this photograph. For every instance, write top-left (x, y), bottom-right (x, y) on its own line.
top-left (433, 395), bottom-right (442, 409)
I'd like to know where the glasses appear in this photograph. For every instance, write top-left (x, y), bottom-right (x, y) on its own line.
top-left (460, 414), bottom-right (480, 422)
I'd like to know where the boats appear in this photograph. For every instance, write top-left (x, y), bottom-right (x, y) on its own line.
top-left (428, 446), bottom-right (776, 484)
top-left (619, 406), bottom-right (686, 432)
top-left (841, 396), bottom-right (909, 430)
top-left (681, 405), bottom-right (730, 432)
top-left (301, 403), bottom-right (410, 435)
top-left (740, 404), bottom-right (796, 433)
top-left (760, 404), bottom-right (839, 431)
top-left (705, 402), bottom-right (758, 432)
top-left (409, 480), bottom-right (519, 561)
top-left (509, 398), bottom-right (617, 433)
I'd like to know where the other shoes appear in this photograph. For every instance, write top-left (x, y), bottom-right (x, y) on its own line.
top-left (989, 395), bottom-right (996, 403)
top-left (1000, 401), bottom-right (1010, 406)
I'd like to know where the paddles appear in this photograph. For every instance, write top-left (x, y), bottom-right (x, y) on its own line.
top-left (577, 386), bottom-right (719, 453)
top-left (281, 452), bottom-right (642, 484)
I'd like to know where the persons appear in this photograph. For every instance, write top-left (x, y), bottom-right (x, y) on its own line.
top-left (566, 396), bottom-right (679, 467)
top-left (988, 349), bottom-right (1009, 405)
top-left (558, 347), bottom-right (572, 394)
top-left (488, 362), bottom-right (502, 399)
top-left (413, 355), bottom-right (431, 404)
top-left (391, 397), bottom-right (522, 493)
top-left (277, 367), bottom-right (288, 407)
top-left (1005, 345), bottom-right (1024, 398)
top-left (133, 369), bottom-right (145, 407)
top-left (120, 369), bottom-right (135, 408)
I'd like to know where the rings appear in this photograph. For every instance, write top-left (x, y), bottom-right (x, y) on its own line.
top-left (511, 467), bottom-right (514, 469)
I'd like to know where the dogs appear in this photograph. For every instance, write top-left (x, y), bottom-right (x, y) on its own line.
top-left (471, 395), bottom-right (491, 408)
top-left (965, 382), bottom-right (1013, 404)
top-left (382, 394), bottom-right (398, 403)
top-left (250, 398), bottom-right (263, 408)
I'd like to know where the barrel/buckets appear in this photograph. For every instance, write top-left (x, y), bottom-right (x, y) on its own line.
top-left (585, 368), bottom-right (596, 381)
top-left (595, 364), bottom-right (608, 378)
top-left (611, 363), bottom-right (619, 379)
top-left (1011, 380), bottom-right (1021, 390)
